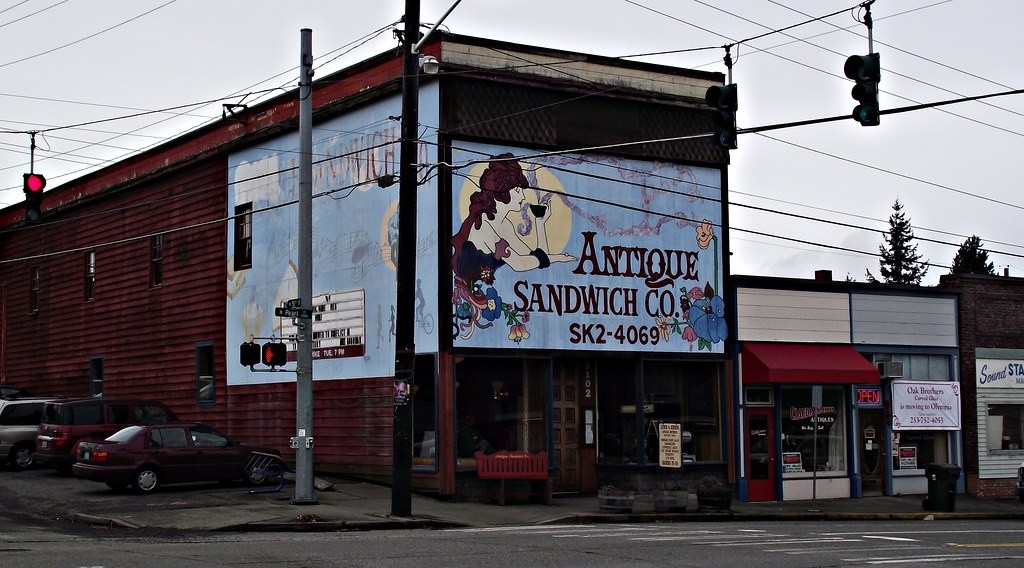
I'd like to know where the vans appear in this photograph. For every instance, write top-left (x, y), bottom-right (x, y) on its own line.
top-left (748, 434), bottom-right (843, 473)
top-left (29, 398), bottom-right (183, 472)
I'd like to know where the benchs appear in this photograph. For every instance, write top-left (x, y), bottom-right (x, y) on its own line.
top-left (475, 449), bottom-right (561, 507)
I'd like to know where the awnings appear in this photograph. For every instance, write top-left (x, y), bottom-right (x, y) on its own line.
top-left (741, 343), bottom-right (880, 383)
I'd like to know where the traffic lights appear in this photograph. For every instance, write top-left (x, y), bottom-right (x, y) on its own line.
top-left (845, 53), bottom-right (881, 126)
top-left (705, 83), bottom-right (737, 151)
top-left (263, 343), bottom-right (287, 366)
top-left (23, 173), bottom-right (48, 224)
top-left (239, 342), bottom-right (261, 367)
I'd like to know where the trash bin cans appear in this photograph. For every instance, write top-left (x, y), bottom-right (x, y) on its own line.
top-left (922, 462), bottom-right (962, 512)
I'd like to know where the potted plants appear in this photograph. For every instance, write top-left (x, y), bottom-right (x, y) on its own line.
top-left (652, 471), bottom-right (689, 513)
top-left (598, 484), bottom-right (635, 514)
top-left (696, 475), bottom-right (733, 513)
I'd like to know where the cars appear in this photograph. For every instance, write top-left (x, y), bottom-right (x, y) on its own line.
top-left (72, 422), bottom-right (284, 497)
top-left (0, 399), bottom-right (71, 472)
